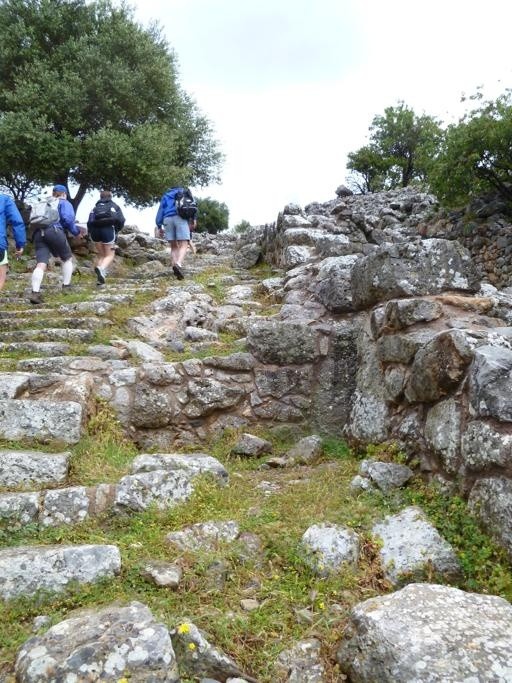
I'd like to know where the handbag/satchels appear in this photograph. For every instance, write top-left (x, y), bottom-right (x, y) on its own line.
top-left (176, 189), bottom-right (198, 218)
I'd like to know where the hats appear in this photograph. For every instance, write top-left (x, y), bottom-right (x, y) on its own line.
top-left (53, 184), bottom-right (67, 193)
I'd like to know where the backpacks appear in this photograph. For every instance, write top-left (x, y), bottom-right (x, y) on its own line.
top-left (93, 200), bottom-right (115, 226)
top-left (30, 194), bottom-right (66, 229)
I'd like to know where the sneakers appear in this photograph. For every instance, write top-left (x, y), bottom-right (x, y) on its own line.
top-left (62, 284), bottom-right (75, 295)
top-left (94, 266), bottom-right (105, 285)
top-left (173, 263), bottom-right (184, 280)
top-left (30, 291), bottom-right (44, 304)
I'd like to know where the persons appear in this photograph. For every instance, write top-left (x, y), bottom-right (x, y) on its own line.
top-left (1, 190), bottom-right (28, 295)
top-left (28, 183), bottom-right (85, 307)
top-left (86, 189), bottom-right (126, 285)
top-left (155, 183), bottom-right (199, 281)
